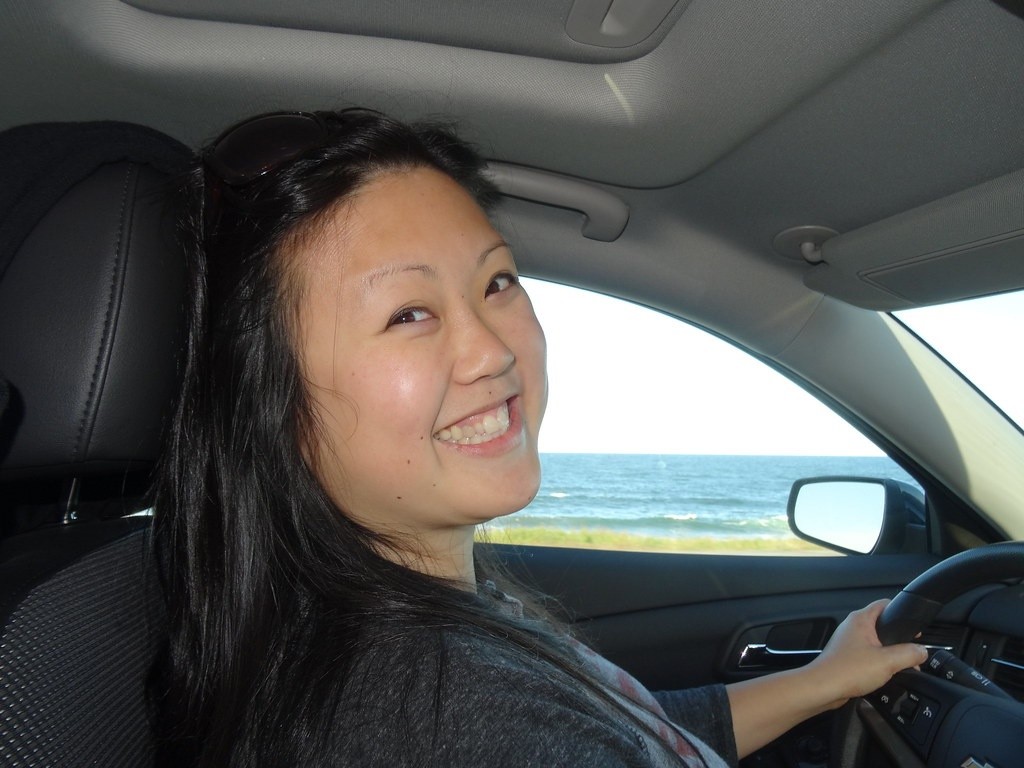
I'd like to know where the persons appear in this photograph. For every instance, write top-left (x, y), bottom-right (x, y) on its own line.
top-left (148, 103), bottom-right (929, 767)
